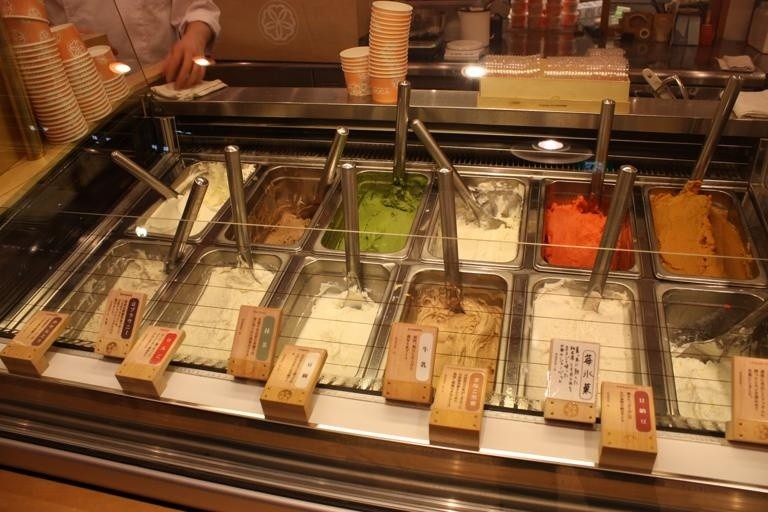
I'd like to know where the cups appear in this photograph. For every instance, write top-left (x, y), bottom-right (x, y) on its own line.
top-left (342, 0), bottom-right (414, 103)
top-left (653, 13), bottom-right (673, 43)
top-left (511, 0), bottom-right (580, 29)
top-left (2, 0), bottom-right (131, 146)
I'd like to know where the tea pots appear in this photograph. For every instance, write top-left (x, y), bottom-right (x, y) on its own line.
top-left (456, 6), bottom-right (496, 48)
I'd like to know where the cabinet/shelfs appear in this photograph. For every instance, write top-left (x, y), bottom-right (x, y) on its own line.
top-left (0, 0), bottom-right (768, 511)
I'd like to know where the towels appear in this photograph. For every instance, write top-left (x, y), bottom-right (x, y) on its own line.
top-left (721, 89), bottom-right (768, 121)
top-left (147, 77), bottom-right (225, 101)
top-left (716, 55), bottom-right (755, 72)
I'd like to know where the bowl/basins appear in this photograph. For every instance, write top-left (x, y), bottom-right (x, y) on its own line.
top-left (412, 10), bottom-right (448, 39)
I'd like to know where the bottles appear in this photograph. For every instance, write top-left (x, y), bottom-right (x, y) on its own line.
top-left (700, 5), bottom-right (714, 46)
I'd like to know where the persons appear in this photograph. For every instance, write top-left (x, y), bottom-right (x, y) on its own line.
top-left (39, 2), bottom-right (224, 91)
